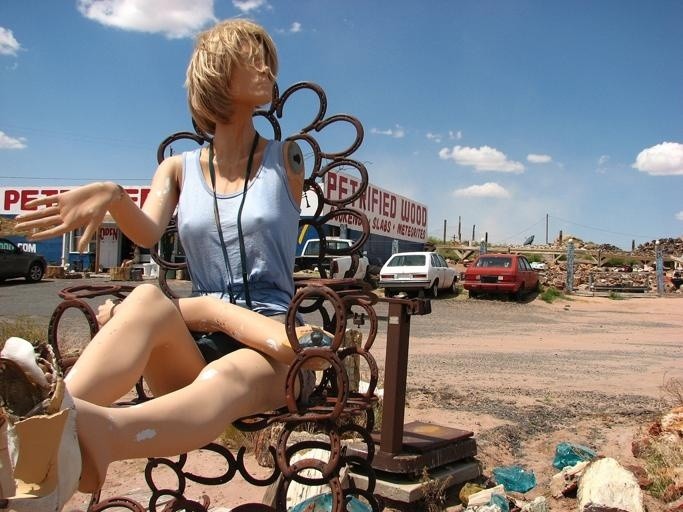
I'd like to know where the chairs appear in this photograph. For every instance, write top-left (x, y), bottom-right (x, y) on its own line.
top-left (47, 81), bottom-right (378, 512)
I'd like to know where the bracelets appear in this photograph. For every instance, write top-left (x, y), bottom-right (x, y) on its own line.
top-left (117, 184), bottom-right (124, 200)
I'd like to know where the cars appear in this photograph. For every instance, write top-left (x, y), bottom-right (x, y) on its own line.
top-left (463, 254), bottom-right (540, 301)
top-left (1, 237), bottom-right (47, 284)
top-left (378, 252), bottom-right (457, 299)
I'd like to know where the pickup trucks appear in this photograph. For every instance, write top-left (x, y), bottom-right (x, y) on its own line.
top-left (293, 236), bottom-right (369, 283)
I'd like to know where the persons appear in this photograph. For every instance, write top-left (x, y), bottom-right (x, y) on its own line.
top-left (0, 17), bottom-right (337, 512)
